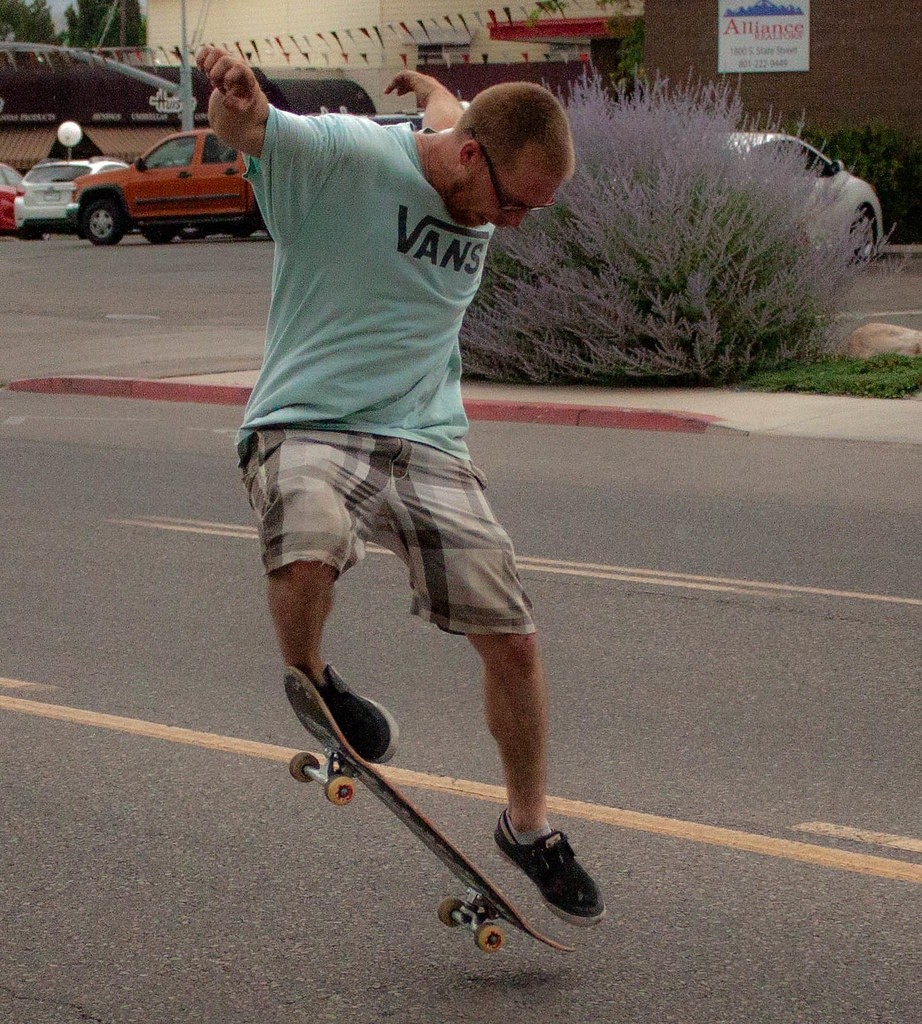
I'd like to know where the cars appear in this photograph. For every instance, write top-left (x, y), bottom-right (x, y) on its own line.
top-left (14, 154), bottom-right (131, 240)
top-left (0, 161), bottom-right (24, 236)
top-left (718, 133), bottom-right (884, 268)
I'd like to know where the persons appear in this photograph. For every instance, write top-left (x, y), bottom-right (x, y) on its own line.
top-left (195, 45), bottom-right (610, 925)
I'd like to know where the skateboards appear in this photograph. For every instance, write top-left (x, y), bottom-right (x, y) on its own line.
top-left (283, 662), bottom-right (578, 956)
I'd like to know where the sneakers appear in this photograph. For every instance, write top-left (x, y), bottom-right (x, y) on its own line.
top-left (314, 664), bottom-right (399, 763)
top-left (493, 809), bottom-right (607, 926)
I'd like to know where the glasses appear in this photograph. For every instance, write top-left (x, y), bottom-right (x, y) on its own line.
top-left (464, 125), bottom-right (557, 213)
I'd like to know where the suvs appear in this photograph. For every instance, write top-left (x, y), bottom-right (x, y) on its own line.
top-left (65, 127), bottom-right (274, 247)
